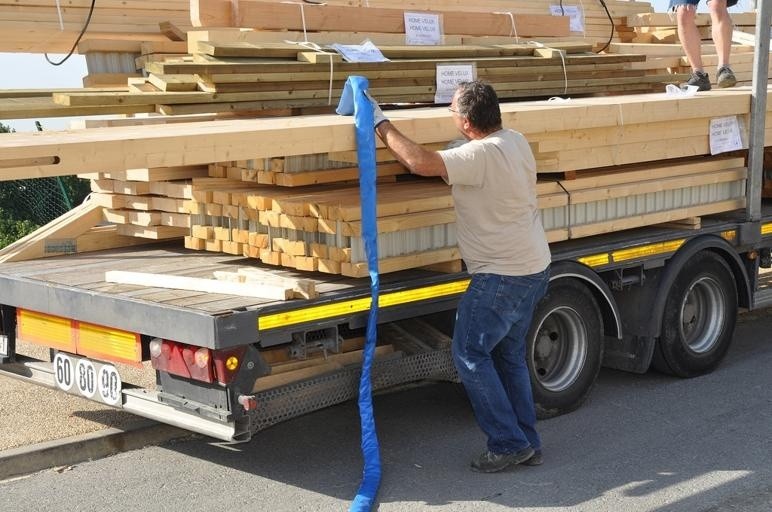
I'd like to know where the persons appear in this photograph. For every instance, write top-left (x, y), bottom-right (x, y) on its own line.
top-left (362, 79), bottom-right (551, 473)
top-left (668, 1), bottom-right (739, 93)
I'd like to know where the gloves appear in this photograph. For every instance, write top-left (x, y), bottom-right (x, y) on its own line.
top-left (363, 89), bottom-right (389, 133)
top-left (447, 139), bottom-right (468, 149)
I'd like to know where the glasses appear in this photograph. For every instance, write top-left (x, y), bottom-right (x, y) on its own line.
top-left (448, 104), bottom-right (466, 118)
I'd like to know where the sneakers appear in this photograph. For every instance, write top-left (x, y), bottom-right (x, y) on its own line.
top-left (678, 72), bottom-right (712, 90)
top-left (470, 446), bottom-right (543, 473)
top-left (716, 67), bottom-right (737, 87)
